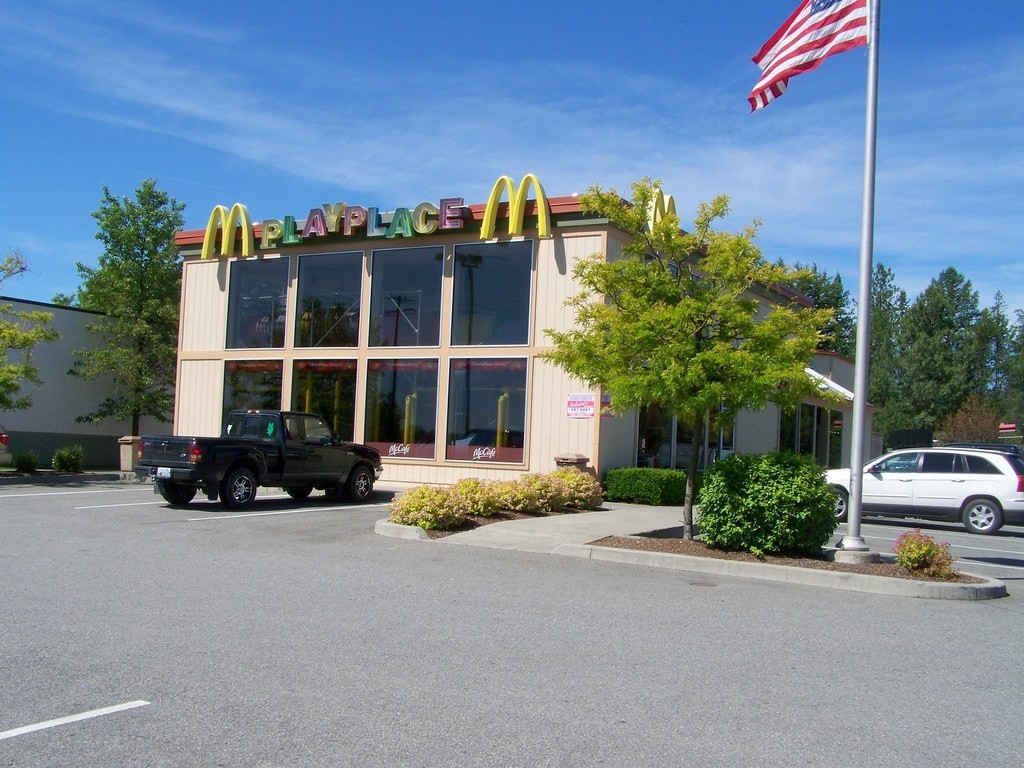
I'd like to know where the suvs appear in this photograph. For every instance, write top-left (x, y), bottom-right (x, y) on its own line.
top-left (818, 442), bottom-right (1024, 534)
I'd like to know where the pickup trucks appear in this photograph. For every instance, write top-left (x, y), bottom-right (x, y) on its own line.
top-left (138, 408), bottom-right (384, 512)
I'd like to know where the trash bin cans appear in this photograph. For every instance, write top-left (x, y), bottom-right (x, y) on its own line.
top-left (117, 435), bottom-right (143, 472)
top-left (553, 453), bottom-right (590, 473)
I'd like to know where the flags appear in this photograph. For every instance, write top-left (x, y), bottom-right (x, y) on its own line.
top-left (747, 0), bottom-right (872, 122)
